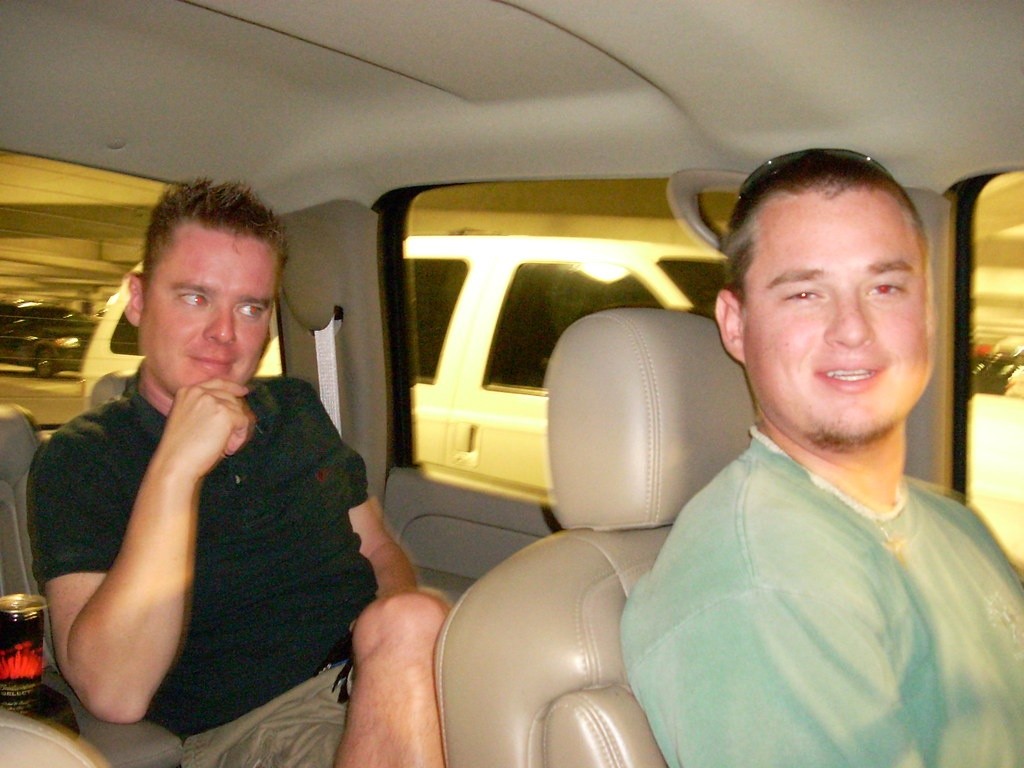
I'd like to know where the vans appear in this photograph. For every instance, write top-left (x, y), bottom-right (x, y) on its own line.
top-left (82, 213), bottom-right (736, 510)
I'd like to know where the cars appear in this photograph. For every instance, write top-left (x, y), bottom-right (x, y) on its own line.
top-left (0, 298), bottom-right (98, 379)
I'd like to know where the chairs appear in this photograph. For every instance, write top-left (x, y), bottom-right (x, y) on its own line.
top-left (432, 308), bottom-right (759, 768)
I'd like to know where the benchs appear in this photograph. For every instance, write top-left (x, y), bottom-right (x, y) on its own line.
top-left (0, 369), bottom-right (186, 768)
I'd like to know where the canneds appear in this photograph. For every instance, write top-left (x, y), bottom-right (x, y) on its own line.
top-left (0, 593), bottom-right (47, 714)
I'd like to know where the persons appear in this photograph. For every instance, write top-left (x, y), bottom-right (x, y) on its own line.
top-left (24, 176), bottom-right (451, 767)
top-left (617, 144), bottom-right (1024, 768)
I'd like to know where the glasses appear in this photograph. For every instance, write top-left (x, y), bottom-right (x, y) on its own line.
top-left (737, 148), bottom-right (896, 195)
